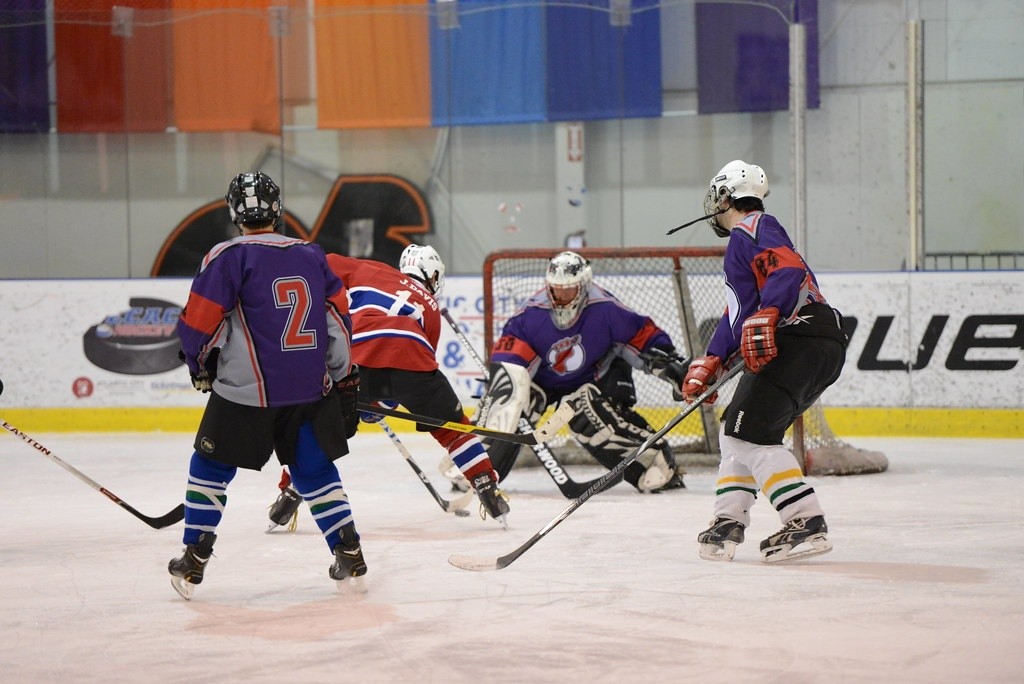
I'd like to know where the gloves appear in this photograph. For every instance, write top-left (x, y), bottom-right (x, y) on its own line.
top-left (333, 363), bottom-right (360, 421)
top-left (682, 355), bottom-right (724, 406)
top-left (740, 307), bottom-right (779, 373)
top-left (191, 377), bottom-right (212, 393)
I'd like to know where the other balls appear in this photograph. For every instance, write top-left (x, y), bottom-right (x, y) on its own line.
top-left (455, 509), bottom-right (470, 517)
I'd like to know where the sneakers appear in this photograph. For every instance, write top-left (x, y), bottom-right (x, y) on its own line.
top-left (264, 483), bottom-right (304, 534)
top-left (471, 471), bottom-right (511, 531)
top-left (167, 532), bottom-right (217, 601)
top-left (697, 517), bottom-right (747, 561)
top-left (329, 525), bottom-right (368, 594)
top-left (760, 515), bottom-right (833, 563)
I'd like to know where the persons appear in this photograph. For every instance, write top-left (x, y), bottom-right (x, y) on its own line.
top-left (268, 244), bottom-right (510, 527)
top-left (682, 160), bottom-right (847, 557)
top-left (167, 172), bottom-right (369, 581)
top-left (438, 250), bottom-right (690, 493)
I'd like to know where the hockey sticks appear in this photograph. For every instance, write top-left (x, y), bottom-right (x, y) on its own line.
top-left (445, 359), bottom-right (746, 573)
top-left (1, 419), bottom-right (187, 531)
top-left (371, 413), bottom-right (476, 512)
top-left (357, 399), bottom-right (580, 446)
top-left (439, 306), bottom-right (625, 500)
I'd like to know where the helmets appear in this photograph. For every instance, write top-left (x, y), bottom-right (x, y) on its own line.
top-left (399, 244), bottom-right (445, 288)
top-left (545, 252), bottom-right (593, 311)
top-left (709, 160), bottom-right (768, 200)
top-left (225, 172), bottom-right (282, 224)
